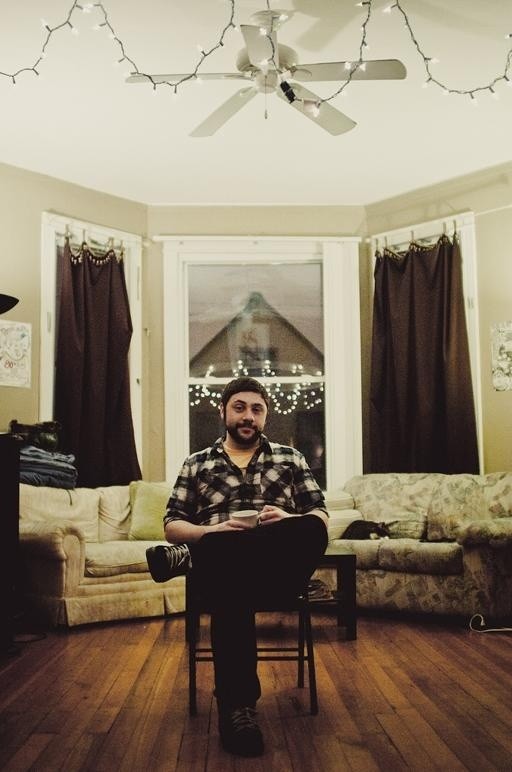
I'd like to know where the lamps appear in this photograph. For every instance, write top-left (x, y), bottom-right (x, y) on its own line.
top-left (176, 569), bottom-right (321, 722)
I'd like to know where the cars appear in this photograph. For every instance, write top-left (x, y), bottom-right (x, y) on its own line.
top-left (230, 509), bottom-right (263, 527)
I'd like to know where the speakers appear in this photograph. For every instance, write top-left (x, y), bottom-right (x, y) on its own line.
top-left (218, 706), bottom-right (264, 758)
top-left (146, 543), bottom-right (192, 583)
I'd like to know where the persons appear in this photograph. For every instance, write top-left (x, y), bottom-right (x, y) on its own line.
top-left (146, 376), bottom-right (329, 758)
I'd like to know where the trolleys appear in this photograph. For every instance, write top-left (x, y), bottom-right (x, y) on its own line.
top-left (125, 9), bottom-right (409, 140)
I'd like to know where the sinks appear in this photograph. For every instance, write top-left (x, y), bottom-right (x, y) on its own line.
top-left (15, 480), bottom-right (189, 623)
top-left (319, 469), bottom-right (512, 628)
